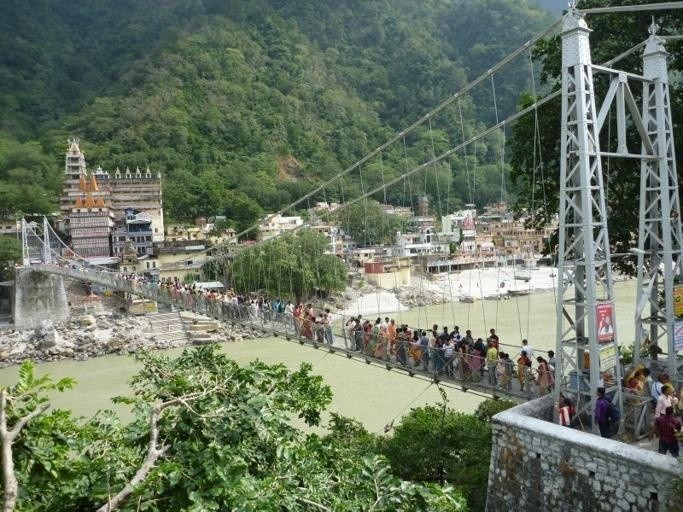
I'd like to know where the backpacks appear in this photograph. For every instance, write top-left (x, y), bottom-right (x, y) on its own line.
top-left (604, 400), bottom-right (620, 424)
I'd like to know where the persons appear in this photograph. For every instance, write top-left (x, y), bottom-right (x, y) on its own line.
top-left (345, 314), bottom-right (556, 398)
top-left (556, 368), bottom-right (683, 458)
top-left (99, 268), bottom-right (334, 351)
top-left (38, 259), bottom-right (85, 276)
